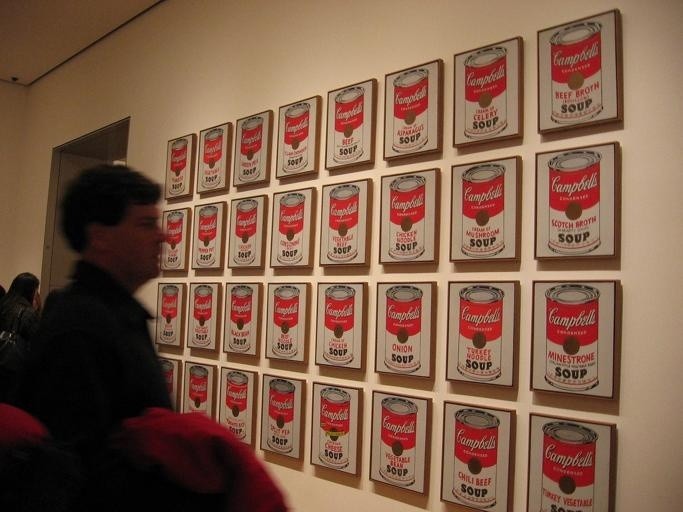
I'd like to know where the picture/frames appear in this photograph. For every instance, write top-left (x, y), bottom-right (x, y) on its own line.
top-left (374, 281), bottom-right (437, 379)
top-left (191, 201), bottom-right (227, 269)
top-left (440, 400), bottom-right (516, 512)
top-left (223, 282), bottom-right (263, 357)
top-left (533, 141), bottom-right (621, 260)
top-left (530, 280), bottom-right (620, 400)
top-left (369, 390), bottom-right (431, 497)
top-left (259, 373), bottom-right (306, 460)
top-left (325, 78), bottom-right (377, 170)
top-left (314, 282), bottom-right (368, 370)
top-left (378, 168), bottom-right (441, 265)
top-left (157, 356), bottom-right (182, 415)
top-left (319, 179), bottom-right (372, 267)
top-left (383, 59), bottom-right (444, 161)
top-left (228, 194), bottom-right (268, 269)
top-left (265, 282), bottom-right (312, 364)
top-left (270, 187), bottom-right (318, 270)
top-left (309, 381), bottom-right (364, 478)
top-left (527, 413), bottom-right (616, 512)
top-left (450, 156), bottom-right (522, 262)
top-left (233, 110), bottom-right (273, 187)
top-left (452, 37), bottom-right (522, 147)
top-left (155, 282), bottom-right (186, 349)
top-left (219, 366), bottom-right (258, 447)
top-left (196, 122), bottom-right (233, 194)
top-left (537, 9), bottom-right (624, 133)
top-left (186, 282), bottom-right (222, 351)
top-left (275, 94), bottom-right (321, 181)
top-left (182, 361), bottom-right (217, 422)
top-left (446, 281), bottom-right (518, 388)
top-left (159, 208), bottom-right (192, 271)
top-left (164, 133), bottom-right (197, 201)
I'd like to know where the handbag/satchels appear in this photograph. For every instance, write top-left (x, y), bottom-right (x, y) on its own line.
top-left (0, 309), bottom-right (27, 359)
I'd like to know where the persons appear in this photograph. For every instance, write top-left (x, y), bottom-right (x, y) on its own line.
top-left (11, 163), bottom-right (175, 441)
top-left (1, 271), bottom-right (43, 369)
